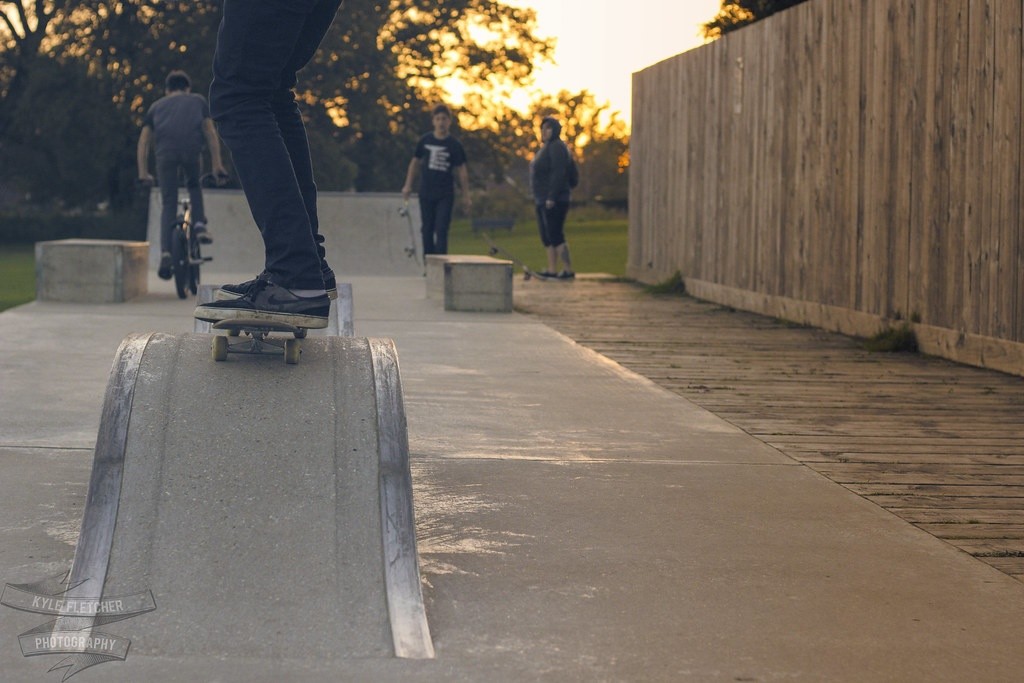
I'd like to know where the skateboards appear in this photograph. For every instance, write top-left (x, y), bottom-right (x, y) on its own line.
top-left (400, 199), bottom-right (423, 269)
top-left (209, 318), bottom-right (308, 366)
top-left (482, 231), bottom-right (547, 282)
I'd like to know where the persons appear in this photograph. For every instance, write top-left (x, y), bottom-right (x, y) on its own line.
top-left (530, 118), bottom-right (575, 279)
top-left (401, 105), bottom-right (472, 276)
top-left (193, 0), bottom-right (343, 327)
top-left (137, 73), bottom-right (228, 280)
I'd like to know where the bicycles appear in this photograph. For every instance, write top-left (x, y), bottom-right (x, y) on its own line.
top-left (134, 169), bottom-right (232, 301)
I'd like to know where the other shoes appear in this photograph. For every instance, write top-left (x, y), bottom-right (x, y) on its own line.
top-left (536, 269), bottom-right (558, 281)
top-left (556, 269), bottom-right (576, 281)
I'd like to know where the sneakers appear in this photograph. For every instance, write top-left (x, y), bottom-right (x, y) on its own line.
top-left (158, 251), bottom-right (174, 280)
top-left (193, 272), bottom-right (331, 329)
top-left (194, 221), bottom-right (213, 245)
top-left (215, 268), bottom-right (338, 301)
